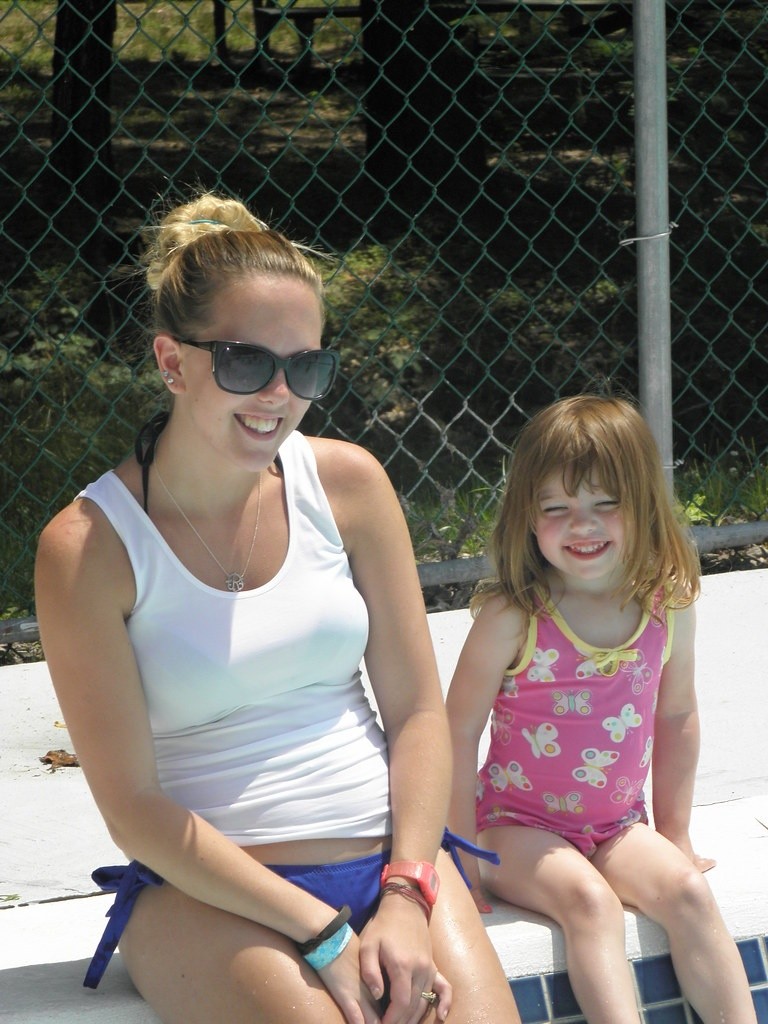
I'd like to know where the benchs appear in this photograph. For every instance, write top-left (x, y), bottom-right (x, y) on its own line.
top-left (259, 6), bottom-right (361, 69)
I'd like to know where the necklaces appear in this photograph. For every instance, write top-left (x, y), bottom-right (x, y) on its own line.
top-left (153, 434), bottom-right (262, 590)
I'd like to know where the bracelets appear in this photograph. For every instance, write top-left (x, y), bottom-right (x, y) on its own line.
top-left (304, 923), bottom-right (352, 971)
top-left (381, 883), bottom-right (430, 917)
top-left (298, 904), bottom-right (352, 954)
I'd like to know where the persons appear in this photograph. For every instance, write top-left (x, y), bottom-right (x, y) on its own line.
top-left (34, 194), bottom-right (526, 1024)
top-left (446, 396), bottom-right (759, 1024)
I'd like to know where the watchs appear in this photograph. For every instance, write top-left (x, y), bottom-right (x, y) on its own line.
top-left (381, 861), bottom-right (440, 902)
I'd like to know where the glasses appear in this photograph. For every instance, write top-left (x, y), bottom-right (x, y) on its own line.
top-left (172, 335), bottom-right (341, 400)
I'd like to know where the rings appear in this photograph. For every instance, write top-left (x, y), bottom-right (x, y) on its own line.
top-left (421, 992), bottom-right (436, 1003)
